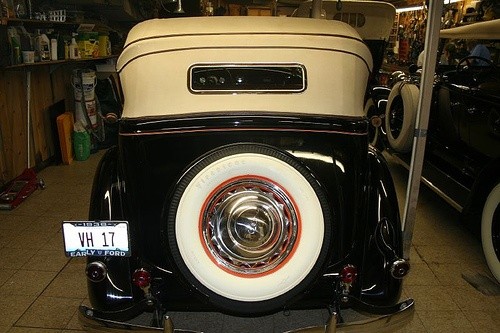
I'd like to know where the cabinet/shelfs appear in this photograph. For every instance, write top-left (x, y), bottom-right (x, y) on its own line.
top-left (5, 18), bottom-right (118, 69)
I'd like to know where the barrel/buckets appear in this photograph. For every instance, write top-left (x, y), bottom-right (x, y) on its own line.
top-left (74, 130), bottom-right (91, 161)
top-left (73, 68), bottom-right (95, 101)
top-left (86, 130), bottom-right (99, 153)
top-left (75, 101), bottom-right (97, 126)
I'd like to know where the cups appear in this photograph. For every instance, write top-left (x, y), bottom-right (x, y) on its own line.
top-left (98, 32), bottom-right (108, 56)
top-left (21, 51), bottom-right (34, 63)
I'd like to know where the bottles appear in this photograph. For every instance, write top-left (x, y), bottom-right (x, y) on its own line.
top-left (26, 0), bottom-right (34, 20)
top-left (10, 34), bottom-right (20, 64)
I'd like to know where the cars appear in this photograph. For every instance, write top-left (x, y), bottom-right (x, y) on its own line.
top-left (62, 16), bottom-right (415, 326)
top-left (365, 18), bottom-right (500, 279)
